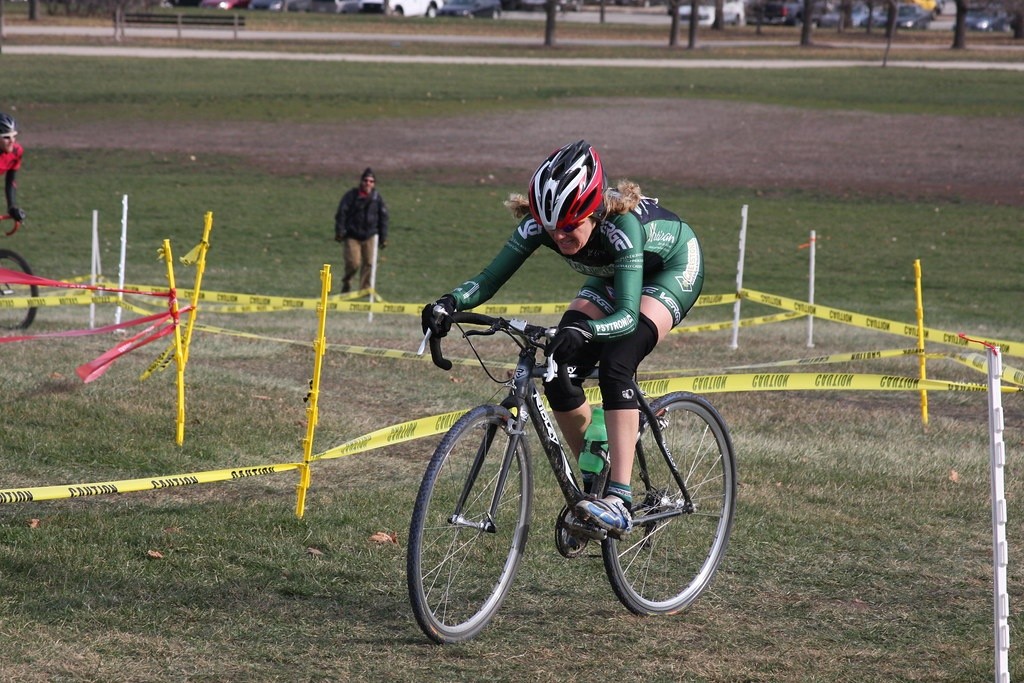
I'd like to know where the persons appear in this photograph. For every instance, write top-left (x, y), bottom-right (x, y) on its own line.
top-left (0, 112), bottom-right (26, 222)
top-left (421, 140), bottom-right (705, 535)
top-left (334, 168), bottom-right (390, 298)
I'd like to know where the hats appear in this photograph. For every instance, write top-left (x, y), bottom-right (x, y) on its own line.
top-left (362, 167), bottom-right (375, 178)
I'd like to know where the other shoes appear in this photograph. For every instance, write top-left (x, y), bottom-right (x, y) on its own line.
top-left (341, 285), bottom-right (350, 294)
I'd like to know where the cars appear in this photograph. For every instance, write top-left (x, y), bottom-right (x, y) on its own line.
top-left (873, 1), bottom-right (930, 30)
top-left (677, 0), bottom-right (746, 27)
top-left (439, 0), bottom-right (502, 19)
top-left (821, 3), bottom-right (870, 29)
top-left (961, 8), bottom-right (1014, 32)
top-left (406, 302), bottom-right (736, 644)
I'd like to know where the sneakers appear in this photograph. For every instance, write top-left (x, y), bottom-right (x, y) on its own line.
top-left (561, 492), bottom-right (595, 548)
top-left (576, 494), bottom-right (633, 542)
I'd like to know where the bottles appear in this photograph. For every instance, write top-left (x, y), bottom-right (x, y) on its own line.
top-left (579, 408), bottom-right (610, 472)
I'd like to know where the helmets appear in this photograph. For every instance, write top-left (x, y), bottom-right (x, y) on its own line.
top-left (528, 139), bottom-right (606, 231)
top-left (0, 111), bottom-right (18, 138)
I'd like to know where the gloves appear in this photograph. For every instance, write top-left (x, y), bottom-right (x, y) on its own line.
top-left (422, 294), bottom-right (457, 339)
top-left (543, 319), bottom-right (593, 365)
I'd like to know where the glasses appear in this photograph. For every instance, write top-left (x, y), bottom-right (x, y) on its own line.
top-left (563, 212), bottom-right (595, 233)
top-left (363, 180), bottom-right (375, 183)
